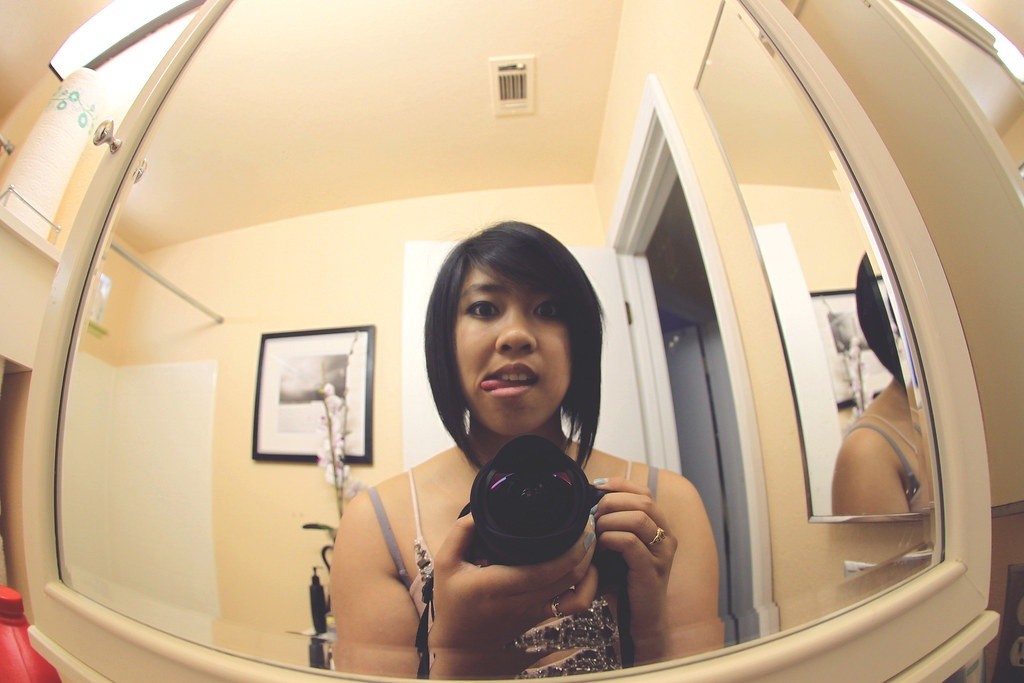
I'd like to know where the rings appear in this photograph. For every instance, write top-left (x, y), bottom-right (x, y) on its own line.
top-left (648, 528), bottom-right (666, 547)
top-left (551, 595), bottom-right (563, 618)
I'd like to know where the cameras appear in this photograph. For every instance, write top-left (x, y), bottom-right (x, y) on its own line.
top-left (456, 434), bottom-right (628, 598)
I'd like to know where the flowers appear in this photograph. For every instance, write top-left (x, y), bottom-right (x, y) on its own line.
top-left (302, 383), bottom-right (364, 542)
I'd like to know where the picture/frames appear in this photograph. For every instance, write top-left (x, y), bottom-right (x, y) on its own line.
top-left (252, 324), bottom-right (377, 465)
top-left (811, 288), bottom-right (894, 410)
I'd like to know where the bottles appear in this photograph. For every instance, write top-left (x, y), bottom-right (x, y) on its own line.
top-left (0, 586), bottom-right (62, 683)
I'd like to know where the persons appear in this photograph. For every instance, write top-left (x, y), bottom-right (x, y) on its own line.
top-left (832, 251), bottom-right (924, 514)
top-left (327, 220), bottom-right (726, 681)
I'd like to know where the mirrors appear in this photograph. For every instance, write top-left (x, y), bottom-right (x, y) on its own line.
top-left (26, 0), bottom-right (994, 683)
top-left (693, 0), bottom-right (934, 523)
